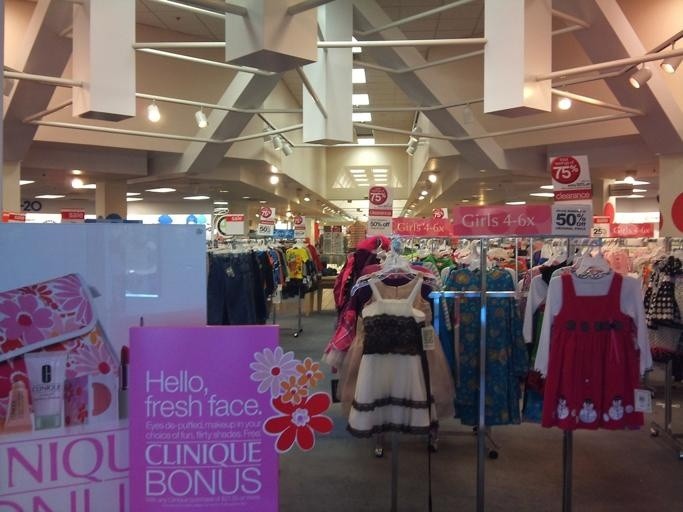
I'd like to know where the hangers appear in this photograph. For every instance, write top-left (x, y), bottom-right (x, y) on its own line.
top-left (461, 240), bottom-right (483, 264)
top-left (351, 241), bottom-right (434, 298)
top-left (543, 238), bottom-right (683, 276)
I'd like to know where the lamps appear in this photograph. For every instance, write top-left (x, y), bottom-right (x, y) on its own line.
top-left (147, 99), bottom-right (293, 157)
top-left (629, 41), bottom-right (682, 89)
top-left (406, 126), bottom-right (422, 156)
top-left (304, 193), bottom-right (310, 202)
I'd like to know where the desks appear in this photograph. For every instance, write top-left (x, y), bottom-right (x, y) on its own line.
top-left (316, 271), bottom-right (340, 312)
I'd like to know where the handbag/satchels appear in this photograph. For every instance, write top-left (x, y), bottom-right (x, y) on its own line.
top-left (1, 273), bottom-right (119, 416)
